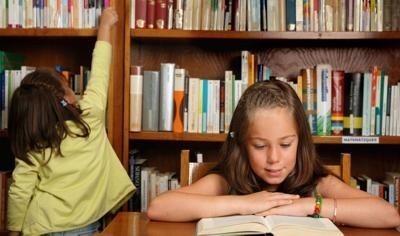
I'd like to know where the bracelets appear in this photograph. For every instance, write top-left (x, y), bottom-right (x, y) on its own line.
top-left (312, 193), bottom-right (337, 224)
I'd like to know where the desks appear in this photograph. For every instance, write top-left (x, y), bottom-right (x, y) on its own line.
top-left (96, 212), bottom-right (400, 236)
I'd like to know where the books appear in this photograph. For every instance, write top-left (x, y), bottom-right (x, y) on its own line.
top-left (1, 1), bottom-right (108, 29)
top-left (324, 153), bottom-right (400, 207)
top-left (195, 215), bottom-right (345, 236)
top-left (1, 171), bottom-right (12, 219)
top-left (131, 0), bottom-right (400, 31)
top-left (0, 50), bottom-right (90, 127)
top-left (129, 51), bottom-right (400, 136)
top-left (129, 147), bottom-right (203, 212)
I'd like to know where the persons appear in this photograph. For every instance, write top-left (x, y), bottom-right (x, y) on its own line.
top-left (147, 78), bottom-right (400, 230)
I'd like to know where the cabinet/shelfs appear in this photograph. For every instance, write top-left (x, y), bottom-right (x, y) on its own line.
top-left (123, 0), bottom-right (400, 214)
top-left (0, 0), bottom-right (122, 235)
